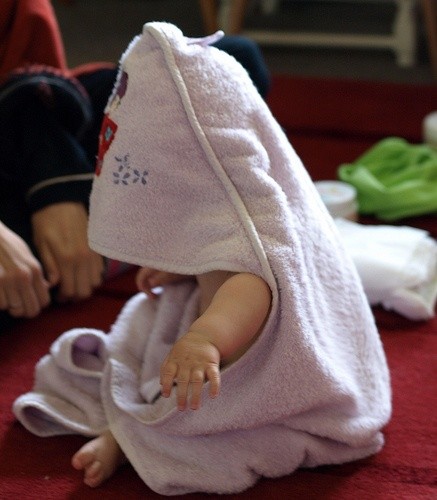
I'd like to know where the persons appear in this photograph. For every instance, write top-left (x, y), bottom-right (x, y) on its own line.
top-left (0, 0), bottom-right (270, 331)
top-left (72, 22), bottom-right (393, 496)
